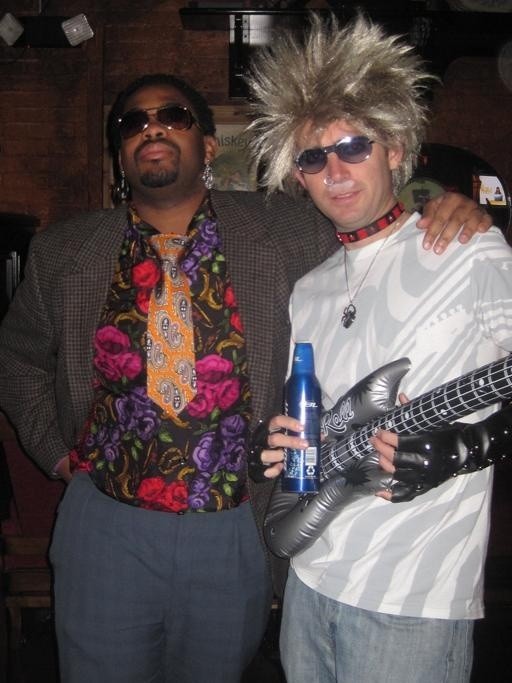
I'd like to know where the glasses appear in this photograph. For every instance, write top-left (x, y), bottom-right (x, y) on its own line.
top-left (294, 136), bottom-right (376, 174)
top-left (118, 103), bottom-right (203, 140)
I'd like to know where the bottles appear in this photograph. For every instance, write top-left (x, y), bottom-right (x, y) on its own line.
top-left (281, 341), bottom-right (321, 494)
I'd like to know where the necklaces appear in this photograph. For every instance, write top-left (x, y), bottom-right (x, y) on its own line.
top-left (337, 216), bottom-right (407, 329)
top-left (336, 199), bottom-right (406, 242)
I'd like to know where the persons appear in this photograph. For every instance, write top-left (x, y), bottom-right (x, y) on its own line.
top-left (493, 186), bottom-right (503, 200)
top-left (238, 8), bottom-right (510, 683)
top-left (5, 69), bottom-right (493, 683)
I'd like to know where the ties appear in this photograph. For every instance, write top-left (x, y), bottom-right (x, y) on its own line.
top-left (147, 234), bottom-right (197, 419)
